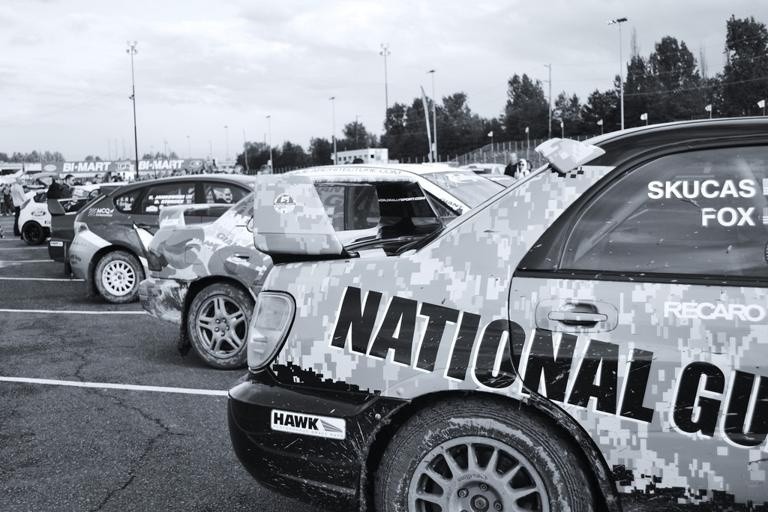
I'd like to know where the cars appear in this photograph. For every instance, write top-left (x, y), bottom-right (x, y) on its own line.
top-left (226, 116), bottom-right (768, 512)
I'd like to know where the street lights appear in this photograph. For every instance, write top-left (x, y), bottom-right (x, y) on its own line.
top-left (125, 41), bottom-right (140, 180)
top-left (377, 44), bottom-right (390, 113)
top-left (543, 64), bottom-right (552, 140)
top-left (428, 68), bottom-right (439, 163)
top-left (329, 96), bottom-right (341, 166)
top-left (609, 16), bottom-right (628, 130)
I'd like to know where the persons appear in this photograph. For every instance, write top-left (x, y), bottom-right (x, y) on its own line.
top-left (0, 176), bottom-right (25, 240)
top-left (90, 168), bottom-right (221, 183)
top-left (59, 174), bottom-right (76, 199)
top-left (514, 158), bottom-right (531, 179)
top-left (504, 153), bottom-right (518, 179)
